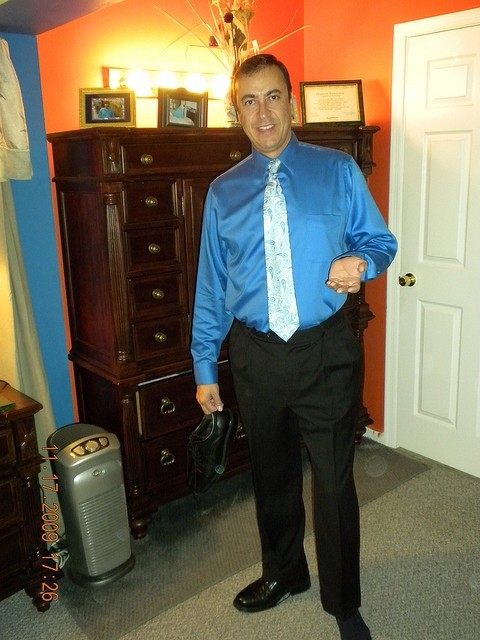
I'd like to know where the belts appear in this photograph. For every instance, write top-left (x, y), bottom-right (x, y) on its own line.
top-left (245, 314), bottom-right (342, 343)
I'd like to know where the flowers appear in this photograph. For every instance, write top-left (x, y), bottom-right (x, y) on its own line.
top-left (153, 0), bottom-right (320, 75)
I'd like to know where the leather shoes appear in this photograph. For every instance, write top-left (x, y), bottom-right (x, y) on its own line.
top-left (233, 576), bottom-right (311, 612)
top-left (189, 408), bottom-right (238, 496)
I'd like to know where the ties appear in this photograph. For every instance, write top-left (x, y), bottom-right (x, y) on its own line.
top-left (263, 159), bottom-right (300, 343)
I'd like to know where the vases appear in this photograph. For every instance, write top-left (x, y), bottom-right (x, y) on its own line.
top-left (225, 82), bottom-right (241, 128)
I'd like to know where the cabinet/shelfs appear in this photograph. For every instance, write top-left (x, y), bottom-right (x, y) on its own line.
top-left (41, 125), bottom-right (381, 542)
top-left (0, 378), bottom-right (64, 613)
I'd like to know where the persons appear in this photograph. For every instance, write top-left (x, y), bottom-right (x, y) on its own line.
top-left (97, 101), bottom-right (114, 119)
top-left (190, 54), bottom-right (398, 640)
top-left (170, 100), bottom-right (184, 119)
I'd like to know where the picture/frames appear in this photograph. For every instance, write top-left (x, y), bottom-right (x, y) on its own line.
top-left (298, 80), bottom-right (366, 127)
top-left (157, 88), bottom-right (208, 128)
top-left (78, 87), bottom-right (137, 128)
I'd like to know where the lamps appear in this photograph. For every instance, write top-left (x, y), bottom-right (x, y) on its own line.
top-left (109, 69), bottom-right (231, 101)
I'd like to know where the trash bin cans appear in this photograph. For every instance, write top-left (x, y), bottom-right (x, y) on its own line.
top-left (46, 424), bottom-right (136, 590)
